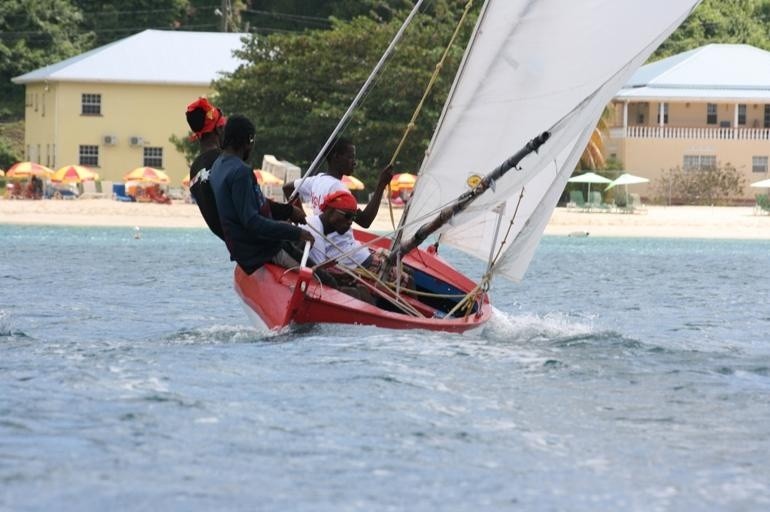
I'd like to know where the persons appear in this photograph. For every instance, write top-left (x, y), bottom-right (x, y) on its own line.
top-left (26, 174), bottom-right (43, 200)
top-left (281, 137), bottom-right (419, 301)
top-left (185, 98), bottom-right (337, 289)
top-left (149, 184), bottom-right (172, 205)
top-left (208, 114), bottom-right (315, 276)
top-left (295, 190), bottom-right (378, 307)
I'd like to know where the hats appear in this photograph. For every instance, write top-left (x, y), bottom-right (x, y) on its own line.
top-left (213, 105), bottom-right (224, 130)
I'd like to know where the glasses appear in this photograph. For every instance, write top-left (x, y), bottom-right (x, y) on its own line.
top-left (334, 207), bottom-right (358, 222)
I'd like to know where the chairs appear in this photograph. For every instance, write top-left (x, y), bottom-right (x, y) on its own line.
top-left (3, 177), bottom-right (134, 203)
top-left (567, 189), bottom-right (650, 215)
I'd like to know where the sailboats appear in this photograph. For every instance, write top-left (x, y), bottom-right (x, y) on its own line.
top-left (233, 0), bottom-right (701, 335)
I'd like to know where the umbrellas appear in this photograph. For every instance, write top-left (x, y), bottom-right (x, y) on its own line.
top-left (121, 167), bottom-right (170, 184)
top-left (0, 161), bottom-right (104, 184)
top-left (253, 169), bottom-right (282, 185)
top-left (341, 174), bottom-right (365, 191)
top-left (750, 179), bottom-right (770, 187)
top-left (387, 172), bottom-right (417, 199)
top-left (568, 172), bottom-right (612, 203)
top-left (613, 174), bottom-right (650, 213)
